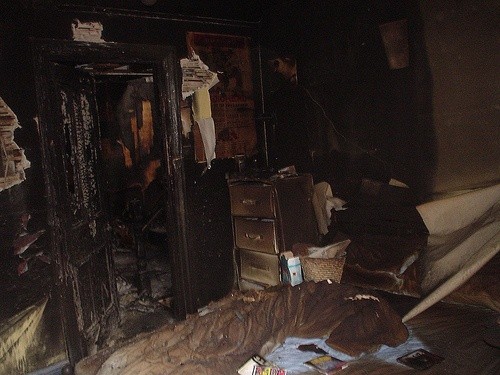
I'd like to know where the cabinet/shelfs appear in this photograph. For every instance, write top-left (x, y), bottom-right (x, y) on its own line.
top-left (226, 171), bottom-right (320, 291)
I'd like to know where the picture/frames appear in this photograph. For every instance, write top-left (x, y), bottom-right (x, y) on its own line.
top-left (176, 22), bottom-right (267, 176)
